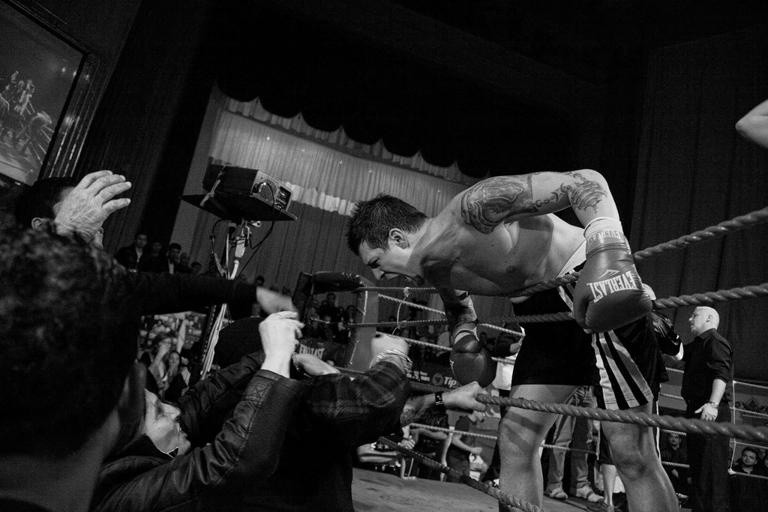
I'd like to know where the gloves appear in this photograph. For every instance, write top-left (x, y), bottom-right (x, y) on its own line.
top-left (573, 215), bottom-right (652, 331)
top-left (450, 321), bottom-right (487, 385)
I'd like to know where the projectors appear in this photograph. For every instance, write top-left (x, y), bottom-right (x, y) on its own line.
top-left (203, 163), bottom-right (294, 212)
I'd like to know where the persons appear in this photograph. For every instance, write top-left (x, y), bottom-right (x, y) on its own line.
top-left (733, 100), bottom-right (768, 148)
top-left (0, 71), bottom-right (52, 157)
